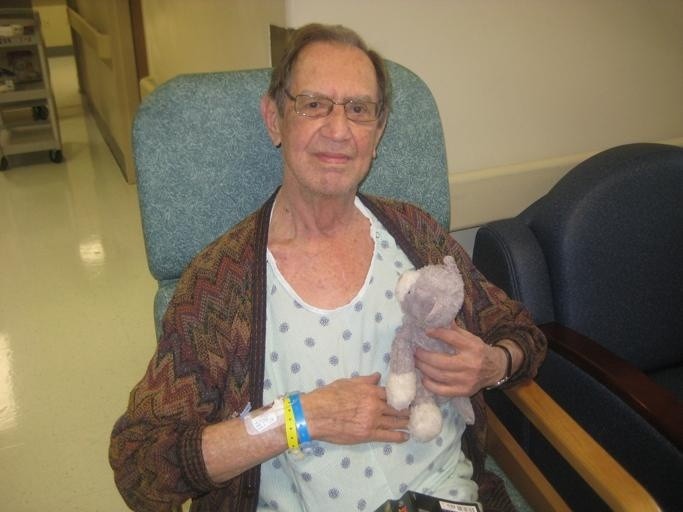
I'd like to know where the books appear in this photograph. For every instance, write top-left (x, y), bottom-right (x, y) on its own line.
top-left (376, 489), bottom-right (483, 512)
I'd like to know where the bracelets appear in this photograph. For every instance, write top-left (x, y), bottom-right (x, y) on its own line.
top-left (280, 391), bottom-right (300, 454)
top-left (286, 394), bottom-right (312, 442)
top-left (485, 343), bottom-right (512, 390)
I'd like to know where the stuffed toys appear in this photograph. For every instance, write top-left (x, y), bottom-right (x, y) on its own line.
top-left (384, 256), bottom-right (476, 441)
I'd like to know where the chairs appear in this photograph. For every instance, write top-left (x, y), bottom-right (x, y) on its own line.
top-left (472, 141), bottom-right (683, 512)
top-left (130, 59), bottom-right (661, 512)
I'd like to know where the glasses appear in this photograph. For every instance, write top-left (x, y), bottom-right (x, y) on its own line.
top-left (277, 81), bottom-right (387, 124)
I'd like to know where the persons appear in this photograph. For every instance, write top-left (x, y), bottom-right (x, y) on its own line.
top-left (107, 22), bottom-right (547, 511)
